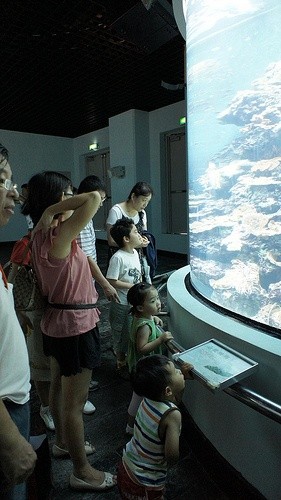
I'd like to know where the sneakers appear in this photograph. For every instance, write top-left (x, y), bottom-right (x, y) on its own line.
top-left (82, 400), bottom-right (95, 413)
top-left (40, 404), bottom-right (55, 429)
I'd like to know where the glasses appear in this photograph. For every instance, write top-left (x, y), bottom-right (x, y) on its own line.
top-left (63, 192), bottom-right (76, 197)
top-left (0, 179), bottom-right (14, 190)
top-left (100, 197), bottom-right (107, 201)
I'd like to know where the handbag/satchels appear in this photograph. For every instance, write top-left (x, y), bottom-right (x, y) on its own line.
top-left (2, 241), bottom-right (40, 311)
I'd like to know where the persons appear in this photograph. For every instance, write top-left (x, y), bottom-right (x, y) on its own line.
top-left (7, 170), bottom-right (194, 500)
top-left (0, 145), bottom-right (38, 499)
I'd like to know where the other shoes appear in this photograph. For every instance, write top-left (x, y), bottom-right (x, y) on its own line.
top-left (114, 362), bottom-right (130, 379)
top-left (89, 380), bottom-right (98, 391)
top-left (70, 467), bottom-right (118, 488)
top-left (125, 423), bottom-right (134, 434)
top-left (52, 440), bottom-right (96, 457)
